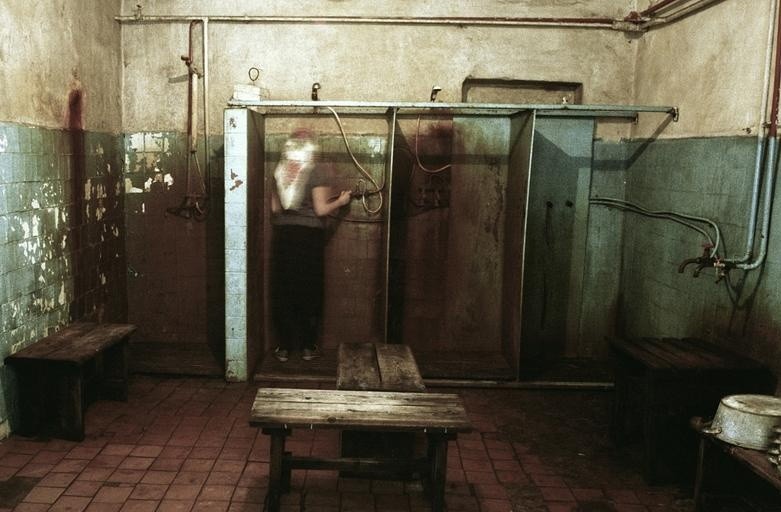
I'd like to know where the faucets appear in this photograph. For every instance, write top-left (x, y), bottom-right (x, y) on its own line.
top-left (693, 244), bottom-right (716, 277)
top-left (678, 244), bottom-right (711, 273)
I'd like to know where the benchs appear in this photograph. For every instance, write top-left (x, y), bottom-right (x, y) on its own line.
top-left (3, 318), bottom-right (138, 442)
top-left (248, 340), bottom-right (472, 507)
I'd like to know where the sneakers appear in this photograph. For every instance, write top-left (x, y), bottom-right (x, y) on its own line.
top-left (274, 347), bottom-right (288, 361)
top-left (302, 345), bottom-right (322, 360)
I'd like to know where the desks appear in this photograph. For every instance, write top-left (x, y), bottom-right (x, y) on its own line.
top-left (602, 328), bottom-right (781, 510)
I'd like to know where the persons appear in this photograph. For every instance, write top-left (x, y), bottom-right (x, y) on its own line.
top-left (271, 127), bottom-right (353, 363)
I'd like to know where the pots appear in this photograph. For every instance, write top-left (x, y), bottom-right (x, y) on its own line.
top-left (700, 393), bottom-right (781, 452)
